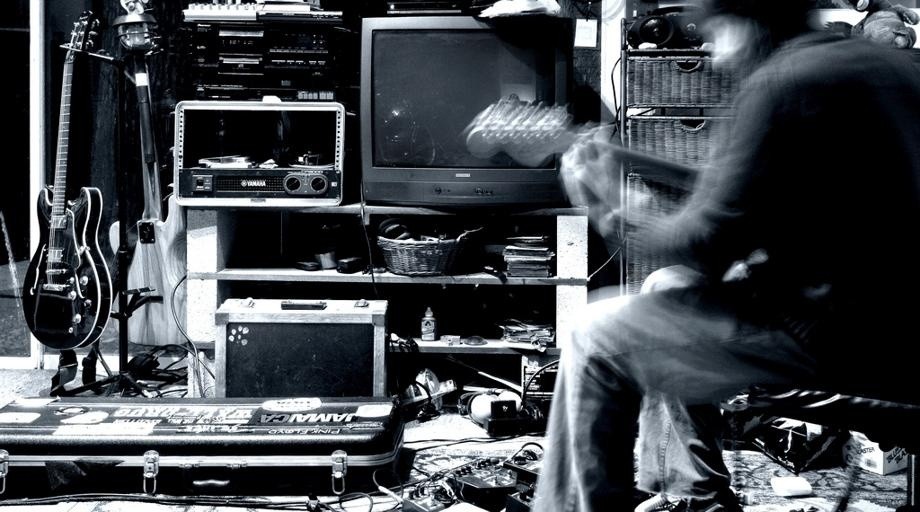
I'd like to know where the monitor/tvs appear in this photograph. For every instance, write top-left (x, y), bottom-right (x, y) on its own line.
top-left (360, 16), bottom-right (573, 208)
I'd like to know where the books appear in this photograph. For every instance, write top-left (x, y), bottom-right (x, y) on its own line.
top-left (501, 321), bottom-right (555, 348)
top-left (501, 232), bottom-right (554, 279)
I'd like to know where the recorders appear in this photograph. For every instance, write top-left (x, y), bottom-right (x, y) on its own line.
top-left (626, 13), bottom-right (707, 49)
top-left (173, 102), bottom-right (346, 206)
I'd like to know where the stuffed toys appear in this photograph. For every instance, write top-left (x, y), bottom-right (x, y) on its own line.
top-left (824, 0), bottom-right (919, 49)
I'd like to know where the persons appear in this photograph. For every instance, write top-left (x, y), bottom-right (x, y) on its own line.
top-left (530, 0), bottom-right (918, 512)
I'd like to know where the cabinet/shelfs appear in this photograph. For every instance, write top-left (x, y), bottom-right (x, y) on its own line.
top-left (615, 13), bottom-right (742, 302)
top-left (179, 193), bottom-right (589, 419)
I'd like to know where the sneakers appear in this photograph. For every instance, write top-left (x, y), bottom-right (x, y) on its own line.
top-left (633, 488), bottom-right (744, 512)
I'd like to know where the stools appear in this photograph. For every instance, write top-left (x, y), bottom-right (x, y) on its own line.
top-left (746, 341), bottom-right (920, 512)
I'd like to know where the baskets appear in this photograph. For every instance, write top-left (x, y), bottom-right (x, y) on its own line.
top-left (377, 227), bottom-right (484, 276)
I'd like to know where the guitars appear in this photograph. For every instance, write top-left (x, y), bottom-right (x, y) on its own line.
top-left (22, 6), bottom-right (115, 350)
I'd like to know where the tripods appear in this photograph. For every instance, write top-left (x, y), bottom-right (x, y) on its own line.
top-left (50, 32), bottom-right (188, 398)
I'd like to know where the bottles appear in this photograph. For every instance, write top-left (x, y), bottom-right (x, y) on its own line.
top-left (421, 306), bottom-right (438, 341)
